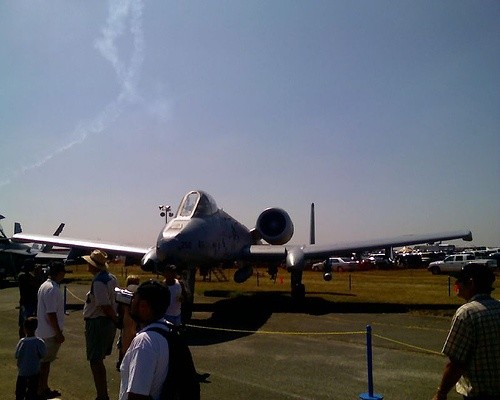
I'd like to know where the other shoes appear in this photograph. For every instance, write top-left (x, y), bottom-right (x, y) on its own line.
top-left (39, 388), bottom-right (61, 397)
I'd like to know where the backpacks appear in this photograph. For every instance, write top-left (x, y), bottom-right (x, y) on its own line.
top-left (141, 320), bottom-right (200, 400)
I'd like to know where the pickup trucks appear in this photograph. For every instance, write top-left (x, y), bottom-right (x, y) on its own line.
top-left (427, 254), bottom-right (497, 275)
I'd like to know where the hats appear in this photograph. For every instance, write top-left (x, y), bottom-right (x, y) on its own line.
top-left (82, 249), bottom-right (109, 270)
top-left (51, 261), bottom-right (64, 272)
top-left (449, 263), bottom-right (495, 290)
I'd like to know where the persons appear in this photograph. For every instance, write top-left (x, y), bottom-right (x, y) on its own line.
top-left (119, 280), bottom-right (199, 400)
top-left (434, 262), bottom-right (500, 400)
top-left (34, 260), bottom-right (65, 400)
top-left (18, 258), bottom-right (42, 339)
top-left (160, 264), bottom-right (190, 327)
top-left (81, 249), bottom-right (119, 400)
top-left (15, 317), bottom-right (48, 400)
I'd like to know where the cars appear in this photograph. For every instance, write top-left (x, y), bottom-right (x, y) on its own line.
top-left (312, 257), bottom-right (358, 272)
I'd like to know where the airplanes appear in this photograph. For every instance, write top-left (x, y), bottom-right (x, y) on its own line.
top-left (11, 190), bottom-right (473, 323)
